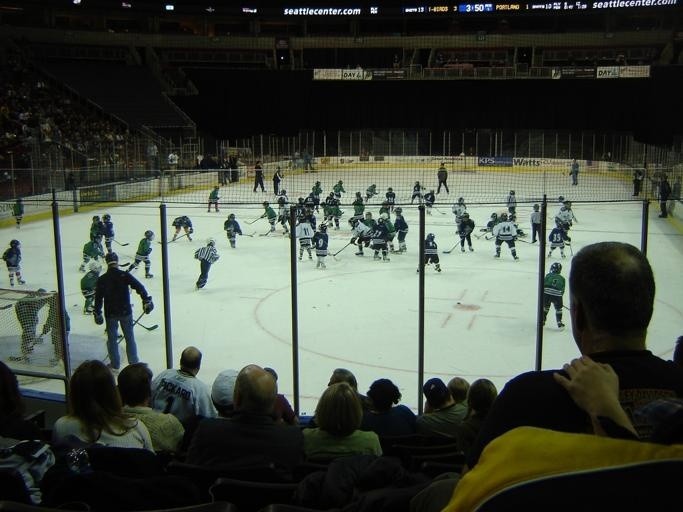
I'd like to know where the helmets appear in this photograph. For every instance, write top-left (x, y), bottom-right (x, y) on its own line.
top-left (315, 180), bottom-right (343, 185)
top-left (227, 213), bottom-right (236, 222)
top-left (550, 263), bottom-right (563, 276)
top-left (93, 214), bottom-right (111, 244)
top-left (491, 213), bottom-right (516, 223)
top-left (278, 197), bottom-right (285, 204)
top-left (105, 252), bottom-right (117, 265)
top-left (90, 262), bottom-right (102, 273)
top-left (144, 230), bottom-right (154, 240)
top-left (457, 197), bottom-right (470, 218)
top-left (298, 192), bottom-right (338, 230)
top-left (263, 201), bottom-right (269, 206)
top-left (426, 233), bottom-right (435, 241)
top-left (206, 240), bottom-right (216, 248)
top-left (349, 207), bottom-right (402, 224)
top-left (11, 240), bottom-right (21, 249)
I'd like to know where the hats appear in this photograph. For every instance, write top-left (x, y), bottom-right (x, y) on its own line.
top-left (422, 378), bottom-right (446, 397)
top-left (356, 192), bottom-right (363, 202)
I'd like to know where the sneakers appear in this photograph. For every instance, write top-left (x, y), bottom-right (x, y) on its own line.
top-left (105, 364), bottom-right (121, 377)
top-left (79, 264), bottom-right (85, 270)
top-left (10, 278), bottom-right (25, 285)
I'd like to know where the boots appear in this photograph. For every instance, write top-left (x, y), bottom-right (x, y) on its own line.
top-left (267, 222), bottom-right (340, 235)
top-left (172, 236), bottom-right (177, 240)
top-left (84, 304), bottom-right (96, 313)
top-left (145, 272), bottom-right (153, 277)
top-left (354, 243), bottom-right (407, 261)
top-left (299, 257), bottom-right (326, 267)
top-left (187, 235), bottom-right (191, 240)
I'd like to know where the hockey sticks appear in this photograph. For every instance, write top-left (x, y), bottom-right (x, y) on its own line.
top-left (244, 217), bottom-right (261, 225)
top-left (435, 206), bottom-right (574, 255)
top-left (157, 233), bottom-right (188, 243)
top-left (328, 243), bottom-right (349, 257)
top-left (241, 231), bottom-right (256, 236)
top-left (104, 256), bottom-right (130, 266)
top-left (134, 320), bottom-right (158, 332)
top-left (259, 222), bottom-right (280, 237)
top-left (113, 239), bottom-right (129, 246)
top-left (8, 333), bottom-right (44, 361)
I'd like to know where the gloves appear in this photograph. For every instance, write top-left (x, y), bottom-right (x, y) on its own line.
top-left (93, 312), bottom-right (103, 324)
top-left (142, 296), bottom-right (154, 313)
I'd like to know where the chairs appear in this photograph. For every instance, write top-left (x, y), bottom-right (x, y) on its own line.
top-left (0, 466), bottom-right (34, 506)
top-left (65, 435), bottom-right (164, 511)
top-left (478, 459), bottom-right (683, 511)
top-left (400, 35), bottom-right (675, 78)
top-left (33, 40), bottom-right (277, 178)
top-left (0, 359), bottom-right (46, 440)
top-left (155, 415), bottom-right (481, 511)
